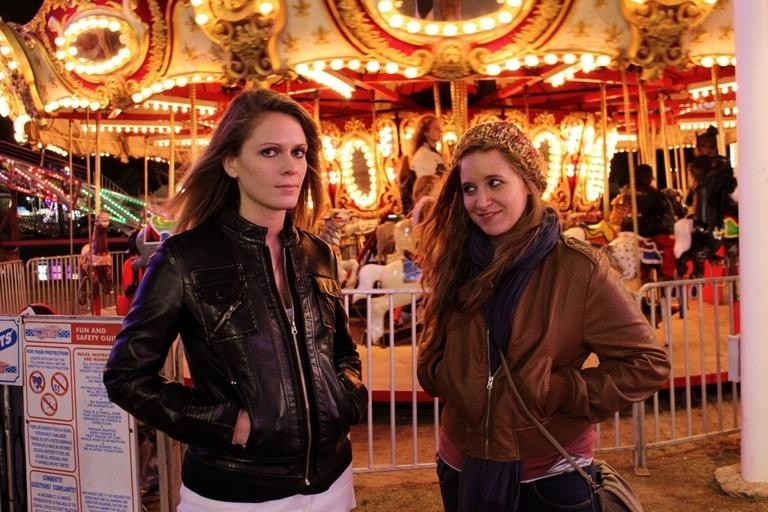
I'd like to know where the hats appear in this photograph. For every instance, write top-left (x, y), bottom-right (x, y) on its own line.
top-left (454, 122), bottom-right (547, 193)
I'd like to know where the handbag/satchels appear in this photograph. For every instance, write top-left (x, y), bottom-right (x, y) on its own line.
top-left (593, 458), bottom-right (642, 512)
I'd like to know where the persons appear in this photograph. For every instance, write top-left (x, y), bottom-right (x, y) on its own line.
top-left (101, 86), bottom-right (365, 511)
top-left (415, 119), bottom-right (672, 512)
top-left (687, 155), bottom-right (737, 234)
top-left (400, 114), bottom-right (446, 221)
top-left (623, 164), bottom-right (670, 238)
